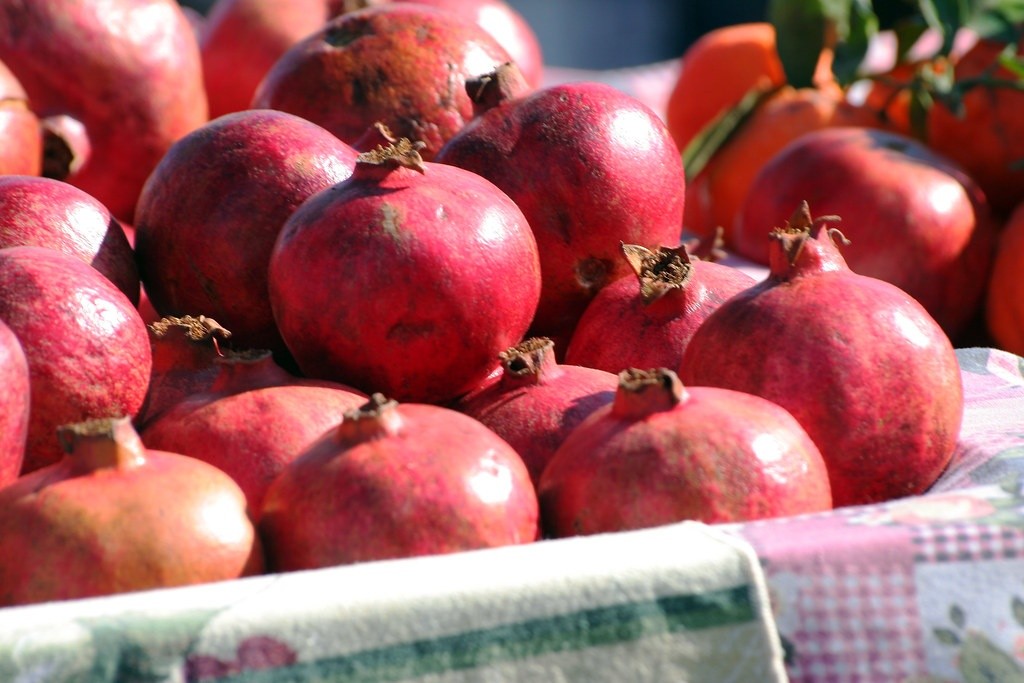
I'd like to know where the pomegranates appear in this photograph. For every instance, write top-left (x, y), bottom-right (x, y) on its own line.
top-left (0, 2), bottom-right (988, 604)
